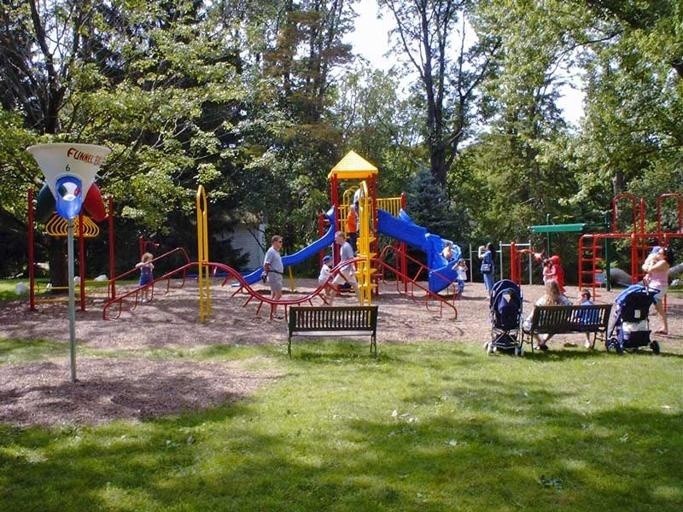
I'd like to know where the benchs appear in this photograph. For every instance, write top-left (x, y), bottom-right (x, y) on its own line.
top-left (525, 305), bottom-right (613, 351)
top-left (287, 304), bottom-right (378, 357)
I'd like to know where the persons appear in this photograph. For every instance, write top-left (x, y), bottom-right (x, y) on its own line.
top-left (641, 246), bottom-right (661, 286)
top-left (316, 254), bottom-right (335, 304)
top-left (541, 258), bottom-right (557, 285)
top-left (523, 280), bottom-right (571, 351)
top-left (353, 181), bottom-right (363, 217)
top-left (639, 250), bottom-right (673, 339)
top-left (259, 234), bottom-right (285, 320)
top-left (449, 257), bottom-right (468, 301)
top-left (346, 205), bottom-right (358, 250)
top-left (572, 288), bottom-right (600, 349)
top-left (547, 255), bottom-right (567, 296)
top-left (325, 230), bottom-right (364, 307)
top-left (133, 251), bottom-right (156, 304)
top-left (436, 241), bottom-right (457, 264)
top-left (476, 241), bottom-right (496, 301)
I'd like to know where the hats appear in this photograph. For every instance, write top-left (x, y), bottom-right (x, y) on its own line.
top-left (323, 255), bottom-right (332, 264)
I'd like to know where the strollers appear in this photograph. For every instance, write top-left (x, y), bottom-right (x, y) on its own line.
top-left (595, 284), bottom-right (660, 355)
top-left (482, 279), bottom-right (523, 356)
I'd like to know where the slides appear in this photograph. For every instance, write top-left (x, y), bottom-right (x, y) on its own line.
top-left (242, 207), bottom-right (462, 293)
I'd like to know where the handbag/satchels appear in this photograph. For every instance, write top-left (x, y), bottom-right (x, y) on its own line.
top-left (481, 264), bottom-right (492, 273)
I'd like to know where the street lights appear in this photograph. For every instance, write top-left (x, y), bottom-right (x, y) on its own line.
top-left (23, 141), bottom-right (110, 387)
